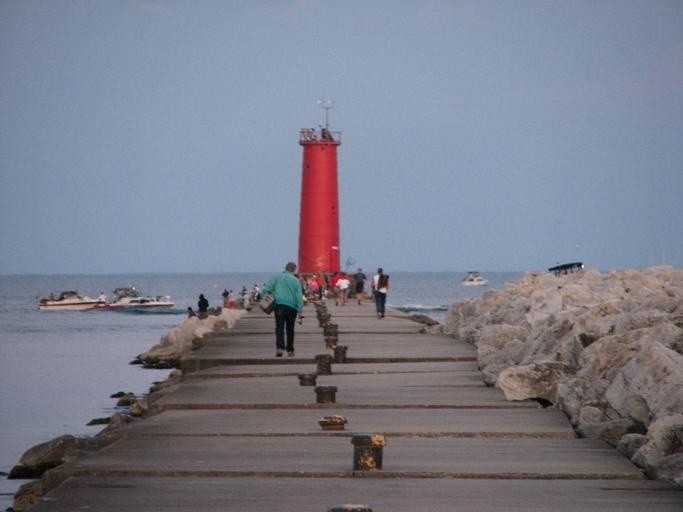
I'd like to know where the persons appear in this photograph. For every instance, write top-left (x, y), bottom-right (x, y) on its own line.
top-left (187, 266), bottom-right (390, 319)
top-left (98, 292), bottom-right (110, 307)
top-left (259, 262), bottom-right (304, 357)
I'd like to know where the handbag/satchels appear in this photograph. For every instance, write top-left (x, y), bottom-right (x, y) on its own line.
top-left (259, 293), bottom-right (275, 315)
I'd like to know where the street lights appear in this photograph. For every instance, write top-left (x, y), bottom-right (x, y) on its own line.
top-left (316, 98), bottom-right (334, 129)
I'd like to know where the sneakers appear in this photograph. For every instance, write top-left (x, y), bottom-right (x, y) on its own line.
top-left (276, 348), bottom-right (283, 357)
top-left (287, 351), bottom-right (295, 357)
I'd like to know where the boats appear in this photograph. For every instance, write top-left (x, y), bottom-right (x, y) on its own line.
top-left (462, 271), bottom-right (489, 286)
top-left (96, 294), bottom-right (174, 310)
top-left (40, 291), bottom-right (99, 311)
